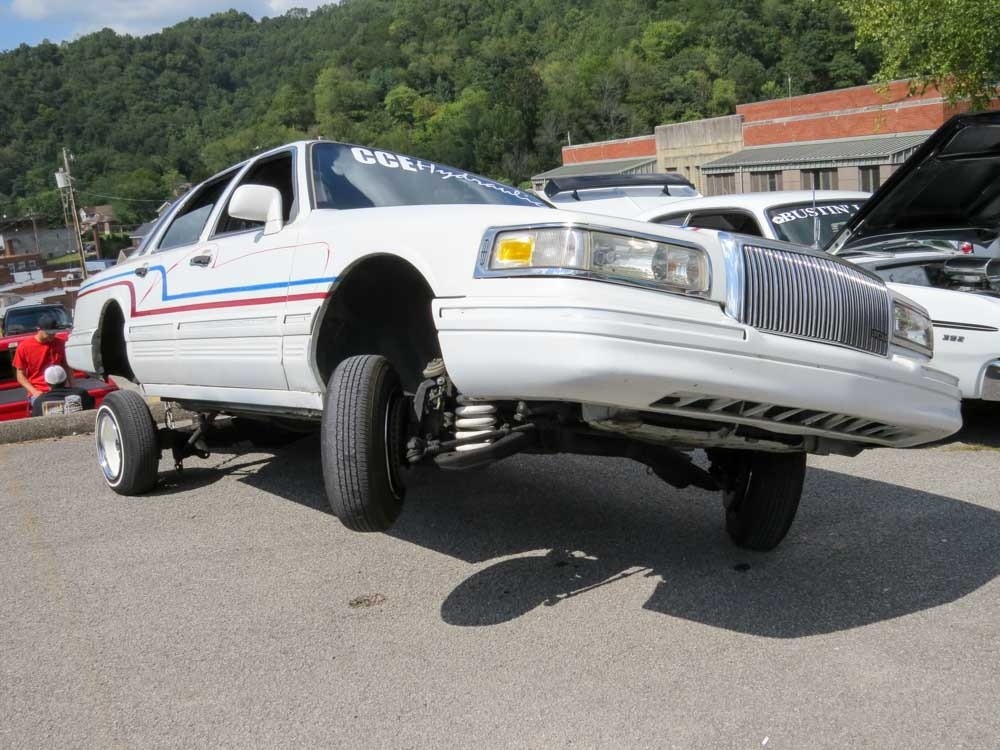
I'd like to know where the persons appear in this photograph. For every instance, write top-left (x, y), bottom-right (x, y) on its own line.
top-left (12, 314), bottom-right (97, 418)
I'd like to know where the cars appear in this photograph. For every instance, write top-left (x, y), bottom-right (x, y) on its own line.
top-left (528, 108), bottom-right (1000, 412)
top-left (2, 301), bottom-right (72, 338)
top-left (60, 135), bottom-right (963, 555)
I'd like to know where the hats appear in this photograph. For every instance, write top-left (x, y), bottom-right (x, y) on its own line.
top-left (39, 315), bottom-right (64, 332)
top-left (44, 366), bottom-right (67, 385)
